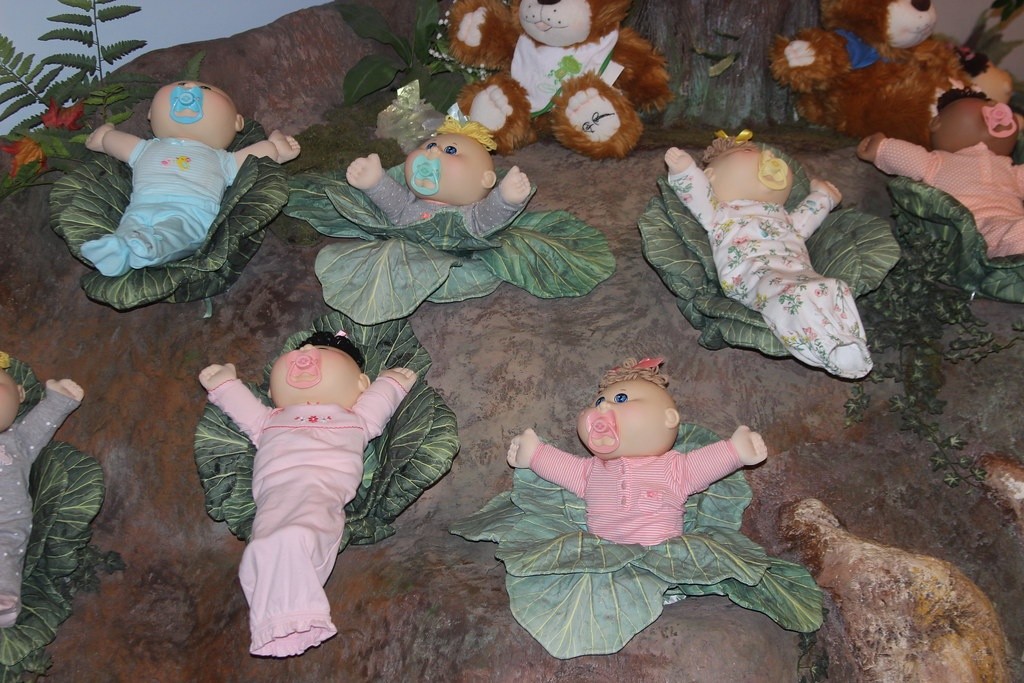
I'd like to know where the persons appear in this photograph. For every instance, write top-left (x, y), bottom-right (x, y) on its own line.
top-left (658, 128), bottom-right (874, 381)
top-left (78, 80), bottom-right (303, 279)
top-left (0, 349), bottom-right (84, 633)
top-left (199, 330), bottom-right (418, 658)
top-left (503, 356), bottom-right (769, 547)
top-left (344, 113), bottom-right (531, 244)
top-left (860, 46), bottom-right (1024, 258)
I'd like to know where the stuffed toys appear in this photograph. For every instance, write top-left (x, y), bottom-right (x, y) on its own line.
top-left (769, 0), bottom-right (974, 157)
top-left (441, 0), bottom-right (675, 162)
